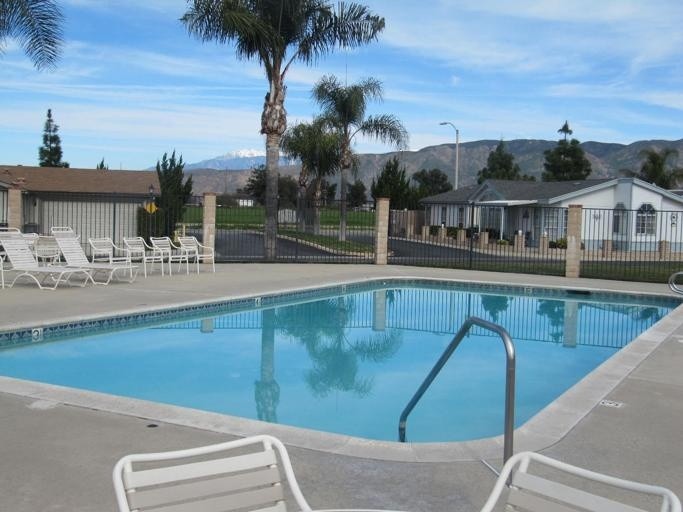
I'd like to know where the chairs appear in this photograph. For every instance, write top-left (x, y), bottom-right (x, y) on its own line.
top-left (1, 226), bottom-right (217, 292)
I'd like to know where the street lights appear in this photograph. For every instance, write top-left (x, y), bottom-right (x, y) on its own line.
top-left (440, 122), bottom-right (458, 190)
top-left (149, 183), bottom-right (155, 237)
